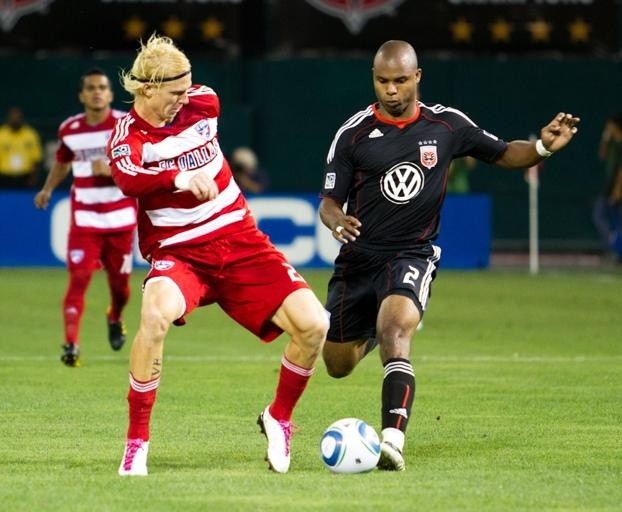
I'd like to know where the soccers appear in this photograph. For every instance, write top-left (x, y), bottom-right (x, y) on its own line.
top-left (321, 418), bottom-right (381, 473)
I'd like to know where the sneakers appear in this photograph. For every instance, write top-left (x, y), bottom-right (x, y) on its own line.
top-left (375, 441), bottom-right (405, 471)
top-left (257, 405), bottom-right (296, 475)
top-left (105, 306), bottom-right (127, 352)
top-left (117, 433), bottom-right (151, 481)
top-left (60, 342), bottom-right (82, 369)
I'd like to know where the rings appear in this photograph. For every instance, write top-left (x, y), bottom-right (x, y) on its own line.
top-left (335, 225), bottom-right (344, 233)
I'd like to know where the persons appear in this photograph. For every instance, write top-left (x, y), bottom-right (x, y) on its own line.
top-left (0, 106), bottom-right (44, 191)
top-left (228, 147), bottom-right (268, 197)
top-left (590, 117), bottom-right (621, 263)
top-left (108, 35), bottom-right (332, 479)
top-left (322, 37), bottom-right (581, 471)
top-left (35, 69), bottom-right (139, 368)
top-left (446, 155), bottom-right (478, 196)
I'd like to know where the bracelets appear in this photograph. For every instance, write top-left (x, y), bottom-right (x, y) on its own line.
top-left (533, 139), bottom-right (554, 160)
top-left (174, 172), bottom-right (199, 191)
